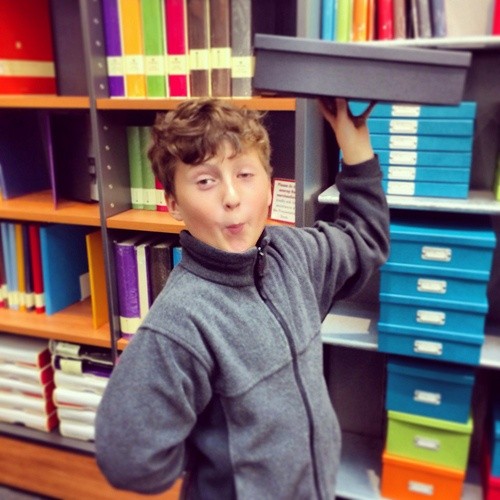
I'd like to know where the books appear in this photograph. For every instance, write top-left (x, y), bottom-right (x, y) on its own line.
top-left (0, 221), bottom-right (109, 329)
top-left (111, 233), bottom-right (183, 339)
top-left (100, 0), bottom-right (274, 97)
top-left (0, 333), bottom-right (112, 443)
top-left (339, 100), bottom-right (476, 197)
top-left (126, 126), bottom-right (168, 212)
top-left (295, 0), bottom-right (446, 42)
top-left (0, 0), bottom-right (57, 96)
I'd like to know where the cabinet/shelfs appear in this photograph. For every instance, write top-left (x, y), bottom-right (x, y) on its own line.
top-left (0, 0), bottom-right (500, 500)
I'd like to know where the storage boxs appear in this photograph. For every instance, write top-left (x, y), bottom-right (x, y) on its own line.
top-left (253, 32), bottom-right (471, 107)
top-left (377, 220), bottom-right (500, 500)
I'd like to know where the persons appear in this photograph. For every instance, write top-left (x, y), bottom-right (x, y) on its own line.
top-left (93, 98), bottom-right (390, 500)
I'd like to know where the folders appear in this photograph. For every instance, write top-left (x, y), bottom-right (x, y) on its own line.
top-left (338, 101), bottom-right (477, 199)
